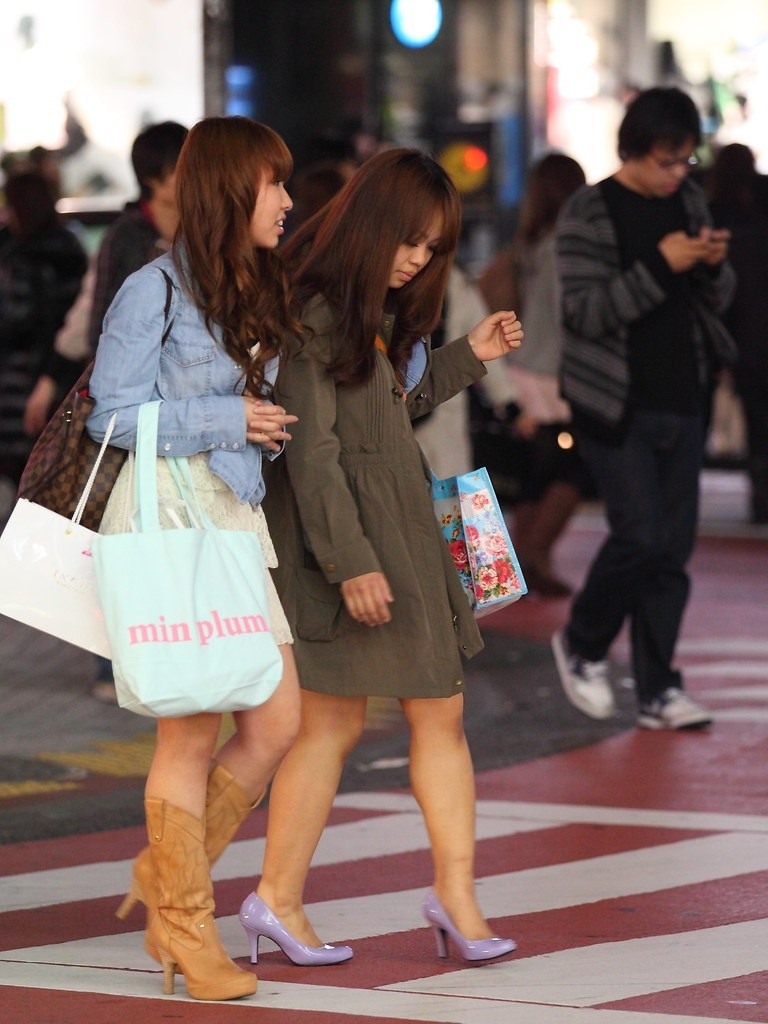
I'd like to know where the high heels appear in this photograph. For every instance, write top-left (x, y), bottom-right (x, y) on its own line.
top-left (424, 893), bottom-right (518, 961)
top-left (238, 891), bottom-right (355, 967)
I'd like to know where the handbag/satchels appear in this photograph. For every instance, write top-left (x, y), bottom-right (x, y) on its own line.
top-left (430, 464), bottom-right (527, 618)
top-left (0, 269), bottom-right (283, 717)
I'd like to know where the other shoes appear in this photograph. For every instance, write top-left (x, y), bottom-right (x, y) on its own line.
top-left (517, 554), bottom-right (571, 599)
top-left (93, 668), bottom-right (118, 703)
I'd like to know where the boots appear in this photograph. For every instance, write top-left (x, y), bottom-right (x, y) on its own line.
top-left (141, 796), bottom-right (257, 1001)
top-left (116, 761), bottom-right (269, 974)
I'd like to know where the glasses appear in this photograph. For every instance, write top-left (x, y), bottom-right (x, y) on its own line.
top-left (644, 145), bottom-right (702, 170)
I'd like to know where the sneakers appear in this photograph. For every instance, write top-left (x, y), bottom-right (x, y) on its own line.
top-left (636, 691), bottom-right (712, 729)
top-left (551, 632), bottom-right (616, 720)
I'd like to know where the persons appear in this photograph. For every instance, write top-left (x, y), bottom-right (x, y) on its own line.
top-left (85, 117), bottom-right (307, 1004)
top-left (409, 261), bottom-right (537, 496)
top-left (239, 145), bottom-right (526, 966)
top-left (549, 85), bottom-right (736, 728)
top-left (700, 143), bottom-right (768, 523)
top-left (324, 116), bottom-right (378, 194)
top-left (506, 152), bottom-right (601, 598)
top-left (0, 122), bottom-right (190, 705)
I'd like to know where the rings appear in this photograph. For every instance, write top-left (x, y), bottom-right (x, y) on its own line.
top-left (261, 432), bottom-right (264, 435)
